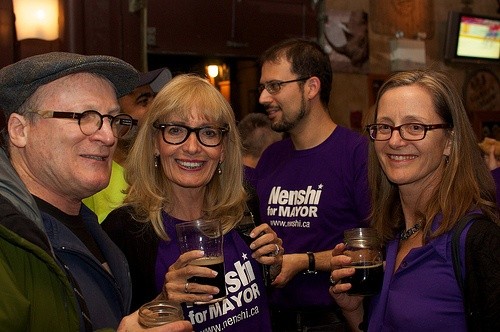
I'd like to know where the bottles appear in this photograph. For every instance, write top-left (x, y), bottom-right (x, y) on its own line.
top-left (339, 227), bottom-right (384, 296)
top-left (138, 300), bottom-right (185, 329)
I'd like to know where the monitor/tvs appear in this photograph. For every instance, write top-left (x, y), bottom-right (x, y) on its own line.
top-left (443, 10), bottom-right (500, 64)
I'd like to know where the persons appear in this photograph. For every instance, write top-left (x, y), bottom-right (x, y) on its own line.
top-left (329, 71), bottom-right (500, 332)
top-left (100, 74), bottom-right (289, 332)
top-left (246, 41), bottom-right (389, 332)
top-left (240, 113), bottom-right (282, 168)
top-left (82, 68), bottom-right (174, 167)
top-left (479, 116), bottom-right (500, 190)
top-left (0, 52), bottom-right (138, 332)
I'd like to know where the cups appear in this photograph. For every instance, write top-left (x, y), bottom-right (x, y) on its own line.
top-left (176, 219), bottom-right (227, 306)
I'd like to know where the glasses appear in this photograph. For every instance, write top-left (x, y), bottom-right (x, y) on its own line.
top-left (153, 122), bottom-right (230, 147)
top-left (17, 108), bottom-right (139, 139)
top-left (257, 76), bottom-right (312, 94)
top-left (366, 123), bottom-right (454, 141)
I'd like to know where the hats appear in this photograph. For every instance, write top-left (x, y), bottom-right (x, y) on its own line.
top-left (135, 67), bottom-right (172, 95)
top-left (0, 51), bottom-right (140, 122)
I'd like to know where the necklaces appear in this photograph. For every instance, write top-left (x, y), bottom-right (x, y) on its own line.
top-left (401, 221), bottom-right (426, 240)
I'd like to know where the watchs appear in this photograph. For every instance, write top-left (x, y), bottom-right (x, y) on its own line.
top-left (303, 252), bottom-right (319, 276)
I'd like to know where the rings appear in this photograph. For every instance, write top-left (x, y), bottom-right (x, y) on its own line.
top-left (184, 282), bottom-right (190, 293)
top-left (330, 276), bottom-right (336, 283)
top-left (275, 244), bottom-right (280, 256)
